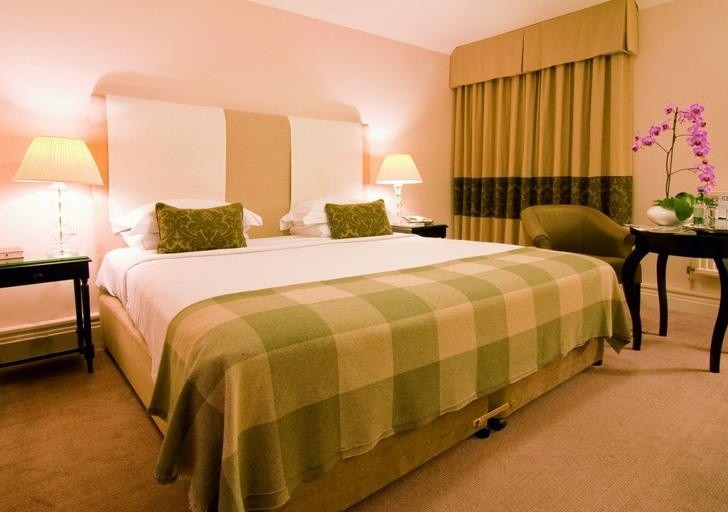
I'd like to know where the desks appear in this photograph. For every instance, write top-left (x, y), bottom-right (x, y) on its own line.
top-left (618, 224), bottom-right (728, 374)
top-left (1, 253), bottom-right (98, 377)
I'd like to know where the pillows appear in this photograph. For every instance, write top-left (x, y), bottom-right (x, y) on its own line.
top-left (125, 192), bottom-right (267, 255)
top-left (275, 187), bottom-right (404, 241)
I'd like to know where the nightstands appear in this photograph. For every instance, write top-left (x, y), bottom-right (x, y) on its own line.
top-left (387, 218), bottom-right (450, 238)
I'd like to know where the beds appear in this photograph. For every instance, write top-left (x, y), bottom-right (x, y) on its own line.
top-left (84, 88), bottom-right (620, 509)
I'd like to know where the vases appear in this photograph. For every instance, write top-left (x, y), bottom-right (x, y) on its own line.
top-left (645, 204), bottom-right (693, 227)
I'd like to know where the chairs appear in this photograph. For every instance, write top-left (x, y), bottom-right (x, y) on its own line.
top-left (517, 204), bottom-right (644, 351)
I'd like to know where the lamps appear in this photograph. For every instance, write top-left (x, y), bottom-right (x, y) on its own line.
top-left (375, 152), bottom-right (422, 221)
top-left (12, 134), bottom-right (105, 257)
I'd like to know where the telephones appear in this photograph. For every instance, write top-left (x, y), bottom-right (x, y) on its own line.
top-left (402, 215), bottom-right (433, 225)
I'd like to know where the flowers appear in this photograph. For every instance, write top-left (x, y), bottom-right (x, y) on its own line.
top-left (628, 97), bottom-right (720, 221)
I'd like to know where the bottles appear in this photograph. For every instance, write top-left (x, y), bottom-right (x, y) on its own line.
top-left (693, 187), bottom-right (728, 229)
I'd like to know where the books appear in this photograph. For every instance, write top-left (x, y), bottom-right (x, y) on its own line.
top-left (0, 247), bottom-right (24, 266)
